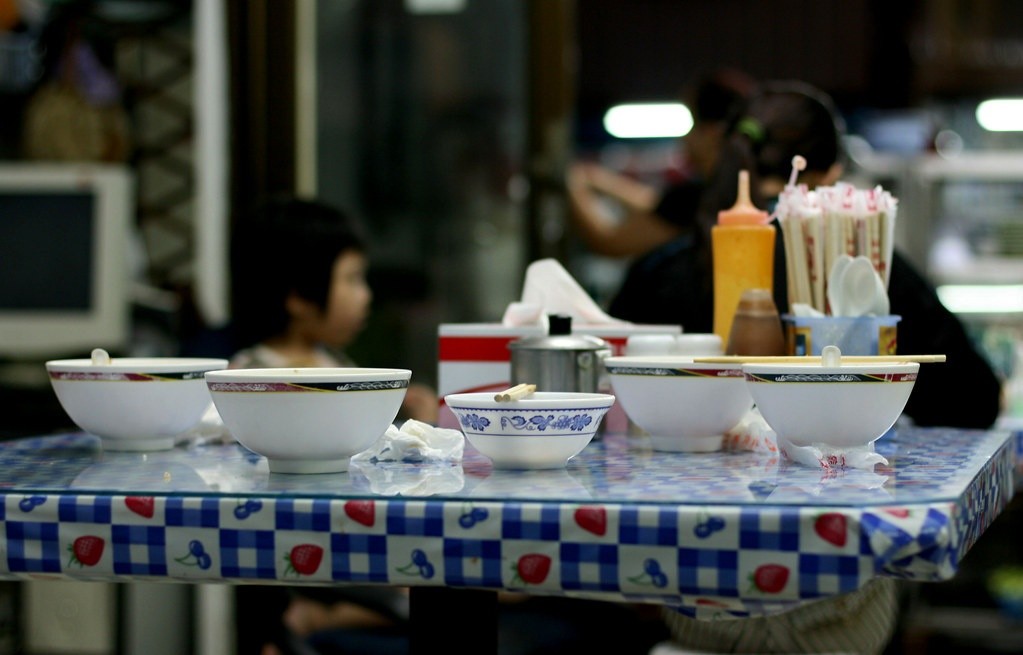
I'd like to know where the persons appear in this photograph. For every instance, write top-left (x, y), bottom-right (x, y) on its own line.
top-left (228, 198), bottom-right (412, 655)
top-left (563, 77), bottom-right (1002, 655)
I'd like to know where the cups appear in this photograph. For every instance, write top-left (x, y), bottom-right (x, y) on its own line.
top-left (626, 334), bottom-right (720, 358)
top-left (781, 315), bottom-right (902, 356)
top-left (508, 316), bottom-right (612, 440)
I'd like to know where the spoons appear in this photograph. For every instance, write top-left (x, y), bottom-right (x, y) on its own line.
top-left (828, 255), bottom-right (891, 317)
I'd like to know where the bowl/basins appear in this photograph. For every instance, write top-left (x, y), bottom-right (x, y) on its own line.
top-left (740, 362), bottom-right (921, 462)
top-left (604, 356), bottom-right (754, 453)
top-left (445, 391), bottom-right (615, 469)
top-left (206, 368), bottom-right (412, 474)
top-left (47, 357), bottom-right (228, 451)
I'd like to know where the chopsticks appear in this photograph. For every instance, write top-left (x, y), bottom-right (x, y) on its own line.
top-left (779, 185), bottom-right (897, 316)
top-left (494, 383), bottom-right (537, 402)
top-left (692, 355), bottom-right (946, 363)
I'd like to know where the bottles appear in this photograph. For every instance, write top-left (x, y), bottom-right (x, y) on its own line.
top-left (727, 288), bottom-right (785, 355)
top-left (712, 170), bottom-right (776, 353)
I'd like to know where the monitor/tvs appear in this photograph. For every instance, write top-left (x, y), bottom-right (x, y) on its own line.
top-left (0, 161), bottom-right (132, 356)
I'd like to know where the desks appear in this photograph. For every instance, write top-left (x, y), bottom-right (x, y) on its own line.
top-left (0, 427), bottom-right (1014, 655)
